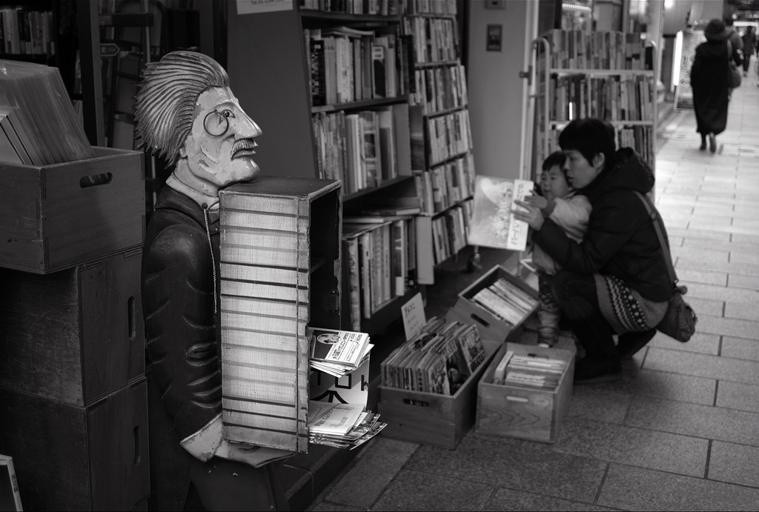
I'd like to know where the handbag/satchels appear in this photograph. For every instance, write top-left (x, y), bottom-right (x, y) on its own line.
top-left (657, 285), bottom-right (699, 343)
top-left (726, 59), bottom-right (741, 87)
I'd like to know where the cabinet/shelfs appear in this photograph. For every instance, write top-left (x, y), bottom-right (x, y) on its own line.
top-left (0, 1), bottom-right (658, 512)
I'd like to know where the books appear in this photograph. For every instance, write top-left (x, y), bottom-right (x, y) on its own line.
top-left (493, 351), bottom-right (567, 392)
top-left (618, 124), bottom-right (656, 171)
top-left (467, 174), bottom-right (534, 251)
top-left (382, 316), bottom-right (486, 394)
top-left (471, 275), bottom-right (539, 329)
top-left (537, 28), bottom-right (654, 70)
top-left (301, 0), bottom-right (480, 332)
top-left (550, 72), bottom-right (655, 123)
top-left (0, 6), bottom-right (54, 54)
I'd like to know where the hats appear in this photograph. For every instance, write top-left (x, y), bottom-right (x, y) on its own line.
top-left (705, 19), bottom-right (734, 42)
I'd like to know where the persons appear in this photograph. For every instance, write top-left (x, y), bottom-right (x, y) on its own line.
top-left (130, 48), bottom-right (264, 510)
top-left (735, 26), bottom-right (744, 37)
top-left (724, 16), bottom-right (744, 61)
top-left (510, 115), bottom-right (677, 384)
top-left (689, 19), bottom-right (734, 153)
top-left (741, 25), bottom-right (756, 77)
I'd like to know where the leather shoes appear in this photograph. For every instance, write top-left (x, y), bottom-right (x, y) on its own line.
top-left (572, 352), bottom-right (622, 382)
top-left (710, 137), bottom-right (716, 153)
top-left (617, 328), bottom-right (659, 362)
